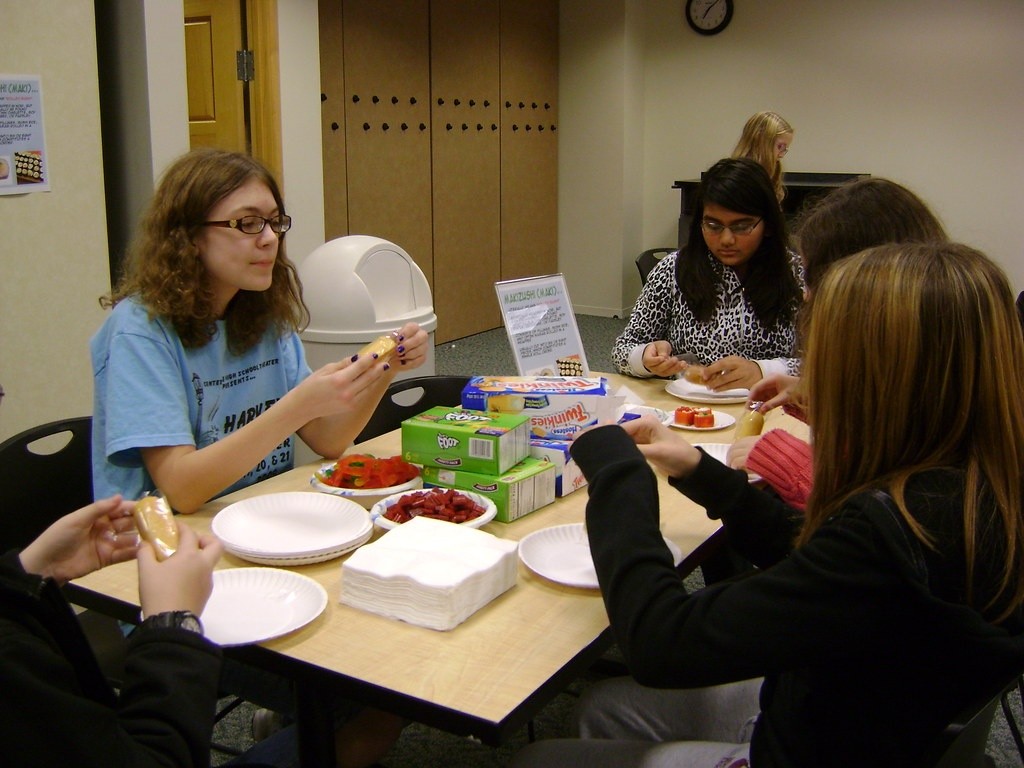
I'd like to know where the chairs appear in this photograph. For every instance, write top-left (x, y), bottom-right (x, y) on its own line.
top-left (636, 246), bottom-right (679, 287)
top-left (923, 289), bottom-right (1024, 768)
top-left (0, 416), bottom-right (247, 757)
top-left (353, 375), bottom-right (471, 446)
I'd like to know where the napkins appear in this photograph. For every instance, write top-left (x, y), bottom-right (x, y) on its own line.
top-left (337, 516), bottom-right (520, 631)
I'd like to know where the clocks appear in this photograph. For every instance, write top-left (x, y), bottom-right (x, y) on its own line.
top-left (686, 0), bottom-right (734, 36)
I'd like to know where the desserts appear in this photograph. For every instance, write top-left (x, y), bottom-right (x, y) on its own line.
top-left (674, 406), bottom-right (715, 428)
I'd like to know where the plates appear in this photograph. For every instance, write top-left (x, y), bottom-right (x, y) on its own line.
top-left (210, 492), bottom-right (375, 566)
top-left (690, 442), bottom-right (761, 484)
top-left (665, 376), bottom-right (750, 405)
top-left (518, 523), bottom-right (684, 590)
top-left (140, 567), bottom-right (328, 648)
top-left (668, 409), bottom-right (737, 431)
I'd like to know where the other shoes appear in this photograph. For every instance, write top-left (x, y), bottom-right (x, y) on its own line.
top-left (251, 710), bottom-right (281, 744)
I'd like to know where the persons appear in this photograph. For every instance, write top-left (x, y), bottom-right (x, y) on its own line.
top-left (569, 179), bottom-right (1024, 768)
top-left (732, 111), bottom-right (793, 203)
top-left (88, 152), bottom-right (427, 515)
top-left (0, 494), bottom-right (226, 768)
top-left (611, 157), bottom-right (804, 392)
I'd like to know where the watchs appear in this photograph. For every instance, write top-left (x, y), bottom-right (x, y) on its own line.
top-left (140, 609), bottom-right (205, 637)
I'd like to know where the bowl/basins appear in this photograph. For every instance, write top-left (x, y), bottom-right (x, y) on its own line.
top-left (309, 475), bottom-right (422, 510)
top-left (369, 488), bottom-right (498, 531)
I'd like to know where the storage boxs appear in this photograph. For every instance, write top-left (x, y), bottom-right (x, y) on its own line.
top-left (400, 375), bottom-right (628, 524)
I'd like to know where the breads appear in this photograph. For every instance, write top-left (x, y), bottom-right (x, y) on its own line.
top-left (735, 411), bottom-right (764, 440)
top-left (355, 335), bottom-right (397, 364)
top-left (683, 365), bottom-right (705, 383)
top-left (133, 496), bottom-right (179, 561)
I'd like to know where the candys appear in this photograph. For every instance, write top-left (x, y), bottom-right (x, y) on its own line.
top-left (313, 454), bottom-right (420, 488)
top-left (383, 487), bottom-right (486, 523)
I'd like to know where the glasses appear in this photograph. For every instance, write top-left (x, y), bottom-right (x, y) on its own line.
top-left (204, 215), bottom-right (291, 234)
top-left (701, 218), bottom-right (763, 235)
top-left (776, 144), bottom-right (788, 154)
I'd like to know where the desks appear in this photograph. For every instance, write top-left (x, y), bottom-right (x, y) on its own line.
top-left (63, 370), bottom-right (817, 768)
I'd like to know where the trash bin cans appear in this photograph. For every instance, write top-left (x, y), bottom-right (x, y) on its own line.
top-left (292, 234), bottom-right (437, 467)
